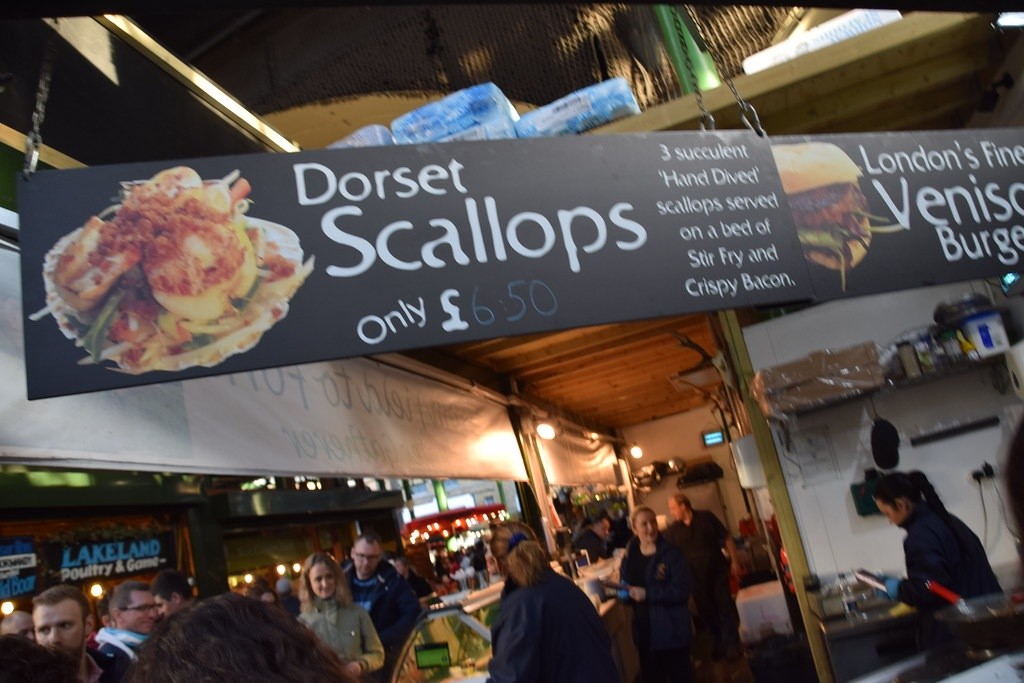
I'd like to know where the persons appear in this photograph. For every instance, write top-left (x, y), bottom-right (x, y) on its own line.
top-left (0, 562), bottom-right (361, 683)
top-left (598, 506), bottom-right (701, 683)
top-left (570, 506), bottom-right (635, 561)
top-left (660, 494), bottom-right (743, 663)
top-left (291, 552), bottom-right (385, 683)
top-left (435, 537), bottom-right (490, 593)
top-left (486, 521), bottom-right (622, 683)
top-left (341, 530), bottom-right (432, 683)
top-left (872, 471), bottom-right (1022, 682)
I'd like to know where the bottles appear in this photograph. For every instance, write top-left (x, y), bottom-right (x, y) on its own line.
top-left (896, 330), bottom-right (964, 378)
top-left (837, 572), bottom-right (864, 626)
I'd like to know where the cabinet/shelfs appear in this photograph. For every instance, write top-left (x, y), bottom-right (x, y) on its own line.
top-left (745, 636), bottom-right (821, 683)
top-left (816, 597), bottom-right (922, 683)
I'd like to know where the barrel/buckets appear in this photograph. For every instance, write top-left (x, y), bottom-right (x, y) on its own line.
top-left (728, 434), bottom-right (767, 489)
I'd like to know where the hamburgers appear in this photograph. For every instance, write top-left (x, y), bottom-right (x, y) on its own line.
top-left (772, 144), bottom-right (904, 294)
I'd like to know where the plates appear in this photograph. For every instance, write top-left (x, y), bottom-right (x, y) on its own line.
top-left (42, 214), bottom-right (304, 375)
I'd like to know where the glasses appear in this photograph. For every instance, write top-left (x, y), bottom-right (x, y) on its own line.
top-left (355, 551), bottom-right (380, 562)
top-left (120, 603), bottom-right (158, 613)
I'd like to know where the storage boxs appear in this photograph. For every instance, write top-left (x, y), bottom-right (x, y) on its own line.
top-left (960, 311), bottom-right (1013, 360)
top-left (751, 341), bottom-right (882, 420)
top-left (735, 578), bottom-right (794, 644)
top-left (742, 9), bottom-right (904, 76)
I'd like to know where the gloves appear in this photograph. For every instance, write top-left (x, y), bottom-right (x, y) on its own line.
top-left (885, 578), bottom-right (902, 604)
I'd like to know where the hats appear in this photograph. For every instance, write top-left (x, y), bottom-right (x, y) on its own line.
top-left (872, 419), bottom-right (900, 469)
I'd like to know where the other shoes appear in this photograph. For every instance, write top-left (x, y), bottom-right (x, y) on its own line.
top-left (711, 640), bottom-right (724, 662)
top-left (726, 644), bottom-right (738, 662)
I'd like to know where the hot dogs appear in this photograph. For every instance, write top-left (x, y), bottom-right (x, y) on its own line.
top-left (50, 174), bottom-right (299, 371)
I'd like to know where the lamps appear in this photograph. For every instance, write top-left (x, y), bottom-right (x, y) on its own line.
top-left (533, 417), bottom-right (564, 440)
